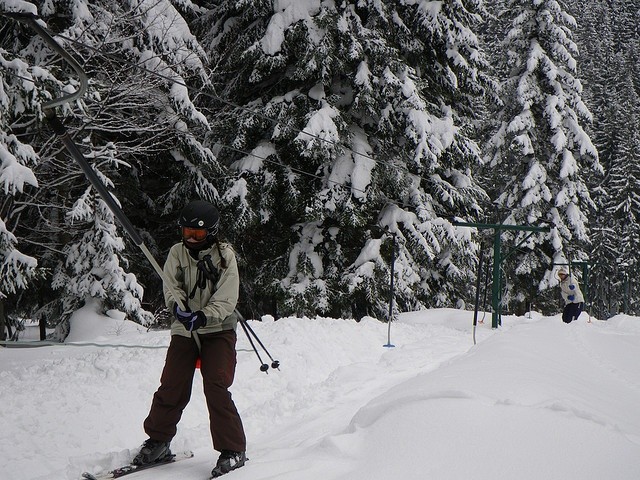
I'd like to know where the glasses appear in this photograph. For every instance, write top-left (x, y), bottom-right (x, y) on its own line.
top-left (181, 220), bottom-right (219, 241)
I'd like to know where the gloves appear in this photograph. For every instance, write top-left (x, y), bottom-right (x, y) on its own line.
top-left (185, 310), bottom-right (207, 331)
top-left (174, 301), bottom-right (193, 324)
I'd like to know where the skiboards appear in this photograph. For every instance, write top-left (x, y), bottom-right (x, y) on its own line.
top-left (82, 450), bottom-right (249, 480)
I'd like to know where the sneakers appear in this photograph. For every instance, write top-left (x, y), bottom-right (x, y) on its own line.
top-left (132, 438), bottom-right (171, 466)
top-left (217, 451), bottom-right (247, 470)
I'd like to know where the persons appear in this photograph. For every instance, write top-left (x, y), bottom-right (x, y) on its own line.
top-left (132, 201), bottom-right (247, 473)
top-left (558, 268), bottom-right (585, 324)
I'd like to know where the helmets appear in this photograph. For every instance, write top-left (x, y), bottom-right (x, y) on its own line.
top-left (180, 200), bottom-right (221, 252)
